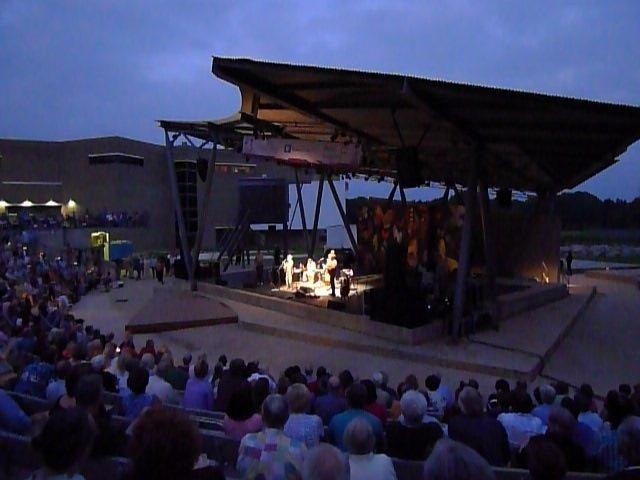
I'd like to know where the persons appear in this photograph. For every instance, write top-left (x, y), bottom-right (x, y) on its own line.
top-left (0, 209), bottom-right (146, 227)
top-left (2, 240), bottom-right (639, 478)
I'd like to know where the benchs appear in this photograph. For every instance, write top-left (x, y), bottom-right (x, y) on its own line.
top-left (1, 373), bottom-right (640, 480)
